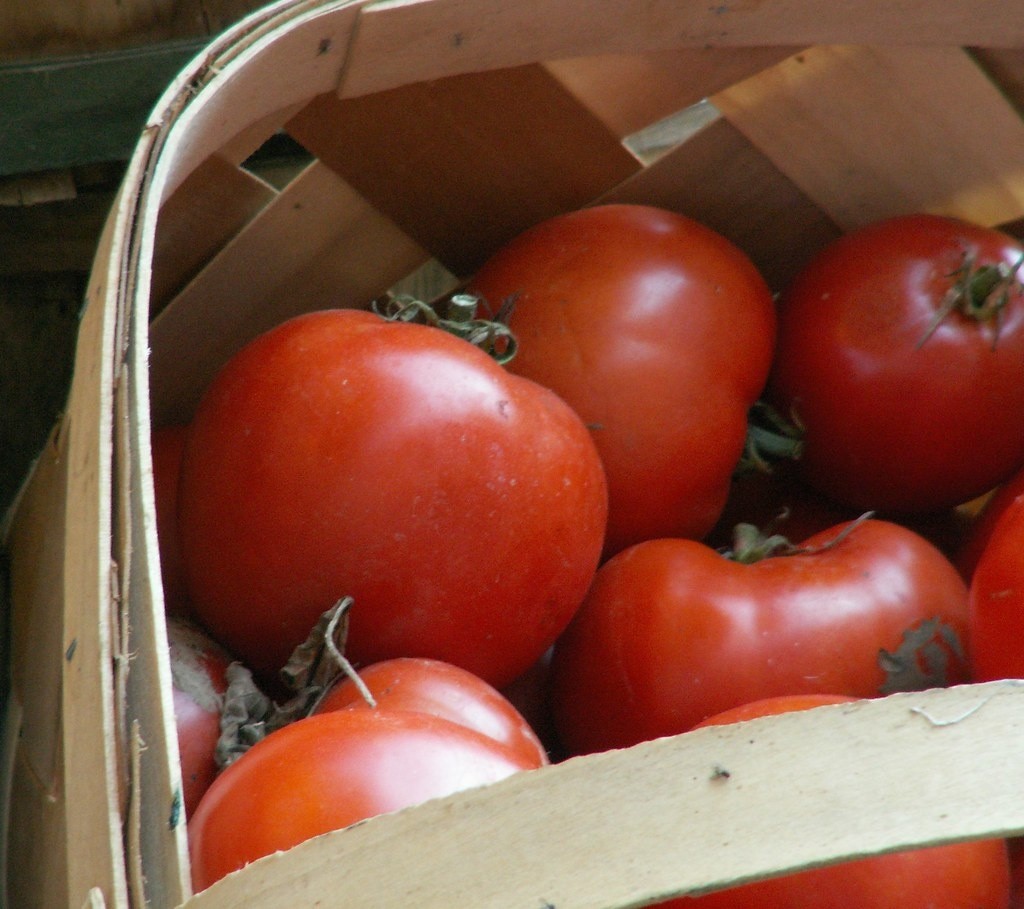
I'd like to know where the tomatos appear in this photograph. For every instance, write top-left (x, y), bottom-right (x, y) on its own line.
top-left (151, 205), bottom-right (1024, 909)
